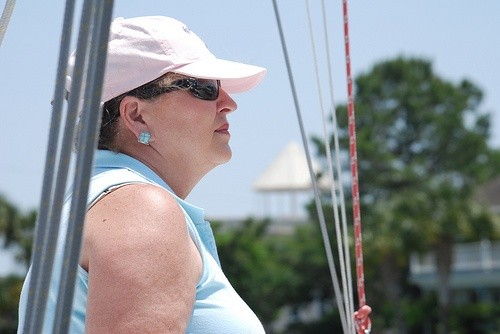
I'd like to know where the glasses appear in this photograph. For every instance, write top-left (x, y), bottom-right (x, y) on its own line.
top-left (129, 77), bottom-right (222, 100)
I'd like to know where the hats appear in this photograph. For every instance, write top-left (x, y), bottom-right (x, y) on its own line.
top-left (65, 16), bottom-right (266, 119)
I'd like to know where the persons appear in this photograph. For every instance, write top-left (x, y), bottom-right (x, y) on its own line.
top-left (17, 16), bottom-right (372, 334)
